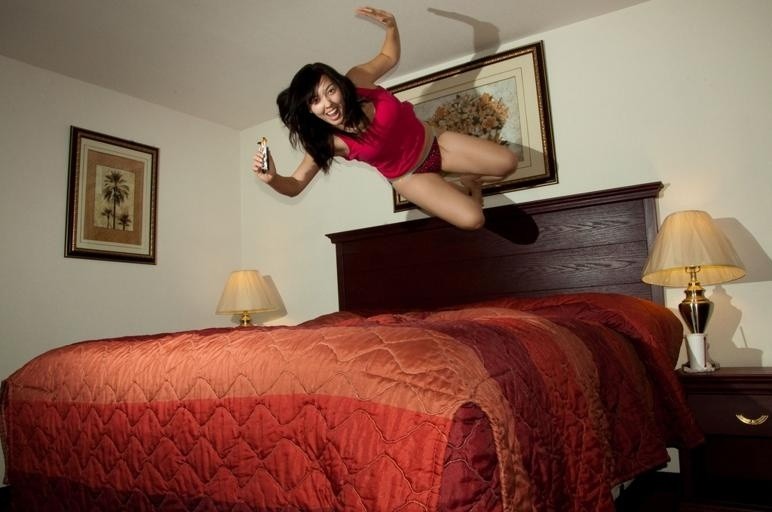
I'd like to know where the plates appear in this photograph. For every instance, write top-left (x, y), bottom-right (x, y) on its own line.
top-left (684, 367), bottom-right (716, 373)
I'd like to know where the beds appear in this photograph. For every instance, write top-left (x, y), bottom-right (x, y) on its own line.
top-left (0, 182), bottom-right (683, 512)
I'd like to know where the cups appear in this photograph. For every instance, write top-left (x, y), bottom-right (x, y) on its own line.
top-left (686, 334), bottom-right (708, 372)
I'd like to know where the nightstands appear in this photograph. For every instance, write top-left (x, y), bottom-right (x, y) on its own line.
top-left (672, 367), bottom-right (771, 511)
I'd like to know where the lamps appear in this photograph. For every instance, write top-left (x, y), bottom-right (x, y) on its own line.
top-left (641, 211), bottom-right (746, 373)
top-left (216, 270), bottom-right (278, 326)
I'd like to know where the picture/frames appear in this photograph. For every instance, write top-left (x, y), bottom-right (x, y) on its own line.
top-left (386, 41), bottom-right (559, 213)
top-left (64, 126), bottom-right (159, 265)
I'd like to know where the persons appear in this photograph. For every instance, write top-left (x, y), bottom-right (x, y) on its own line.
top-left (251, 7), bottom-right (517, 231)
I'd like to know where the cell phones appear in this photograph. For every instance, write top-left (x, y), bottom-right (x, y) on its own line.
top-left (259, 138), bottom-right (267, 171)
